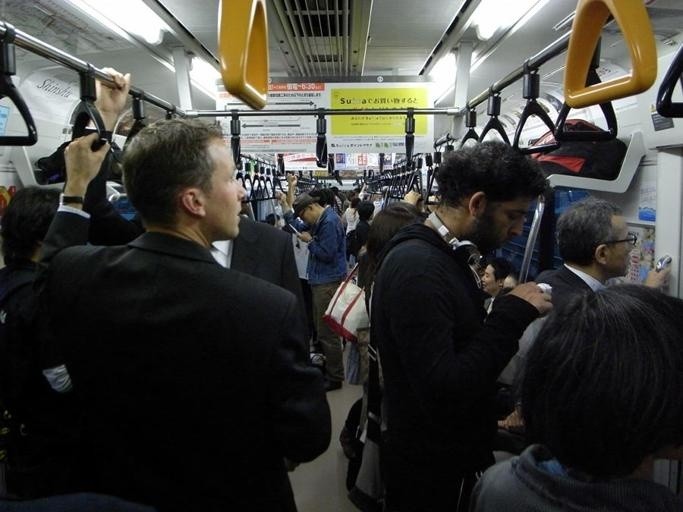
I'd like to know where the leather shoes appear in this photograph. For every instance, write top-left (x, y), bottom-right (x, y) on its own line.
top-left (322, 380), bottom-right (341, 392)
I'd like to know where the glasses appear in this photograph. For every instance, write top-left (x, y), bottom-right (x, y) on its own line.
top-left (593, 232), bottom-right (637, 250)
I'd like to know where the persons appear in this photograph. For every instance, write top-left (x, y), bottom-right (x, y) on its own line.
top-left (2, 68), bottom-right (682, 512)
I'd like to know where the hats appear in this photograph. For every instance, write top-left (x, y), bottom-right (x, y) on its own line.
top-left (292, 192), bottom-right (320, 219)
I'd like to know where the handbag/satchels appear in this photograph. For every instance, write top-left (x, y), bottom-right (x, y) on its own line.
top-left (528, 119), bottom-right (628, 179)
top-left (347, 413), bottom-right (385, 511)
top-left (322, 280), bottom-right (370, 343)
top-left (308, 351), bottom-right (325, 379)
